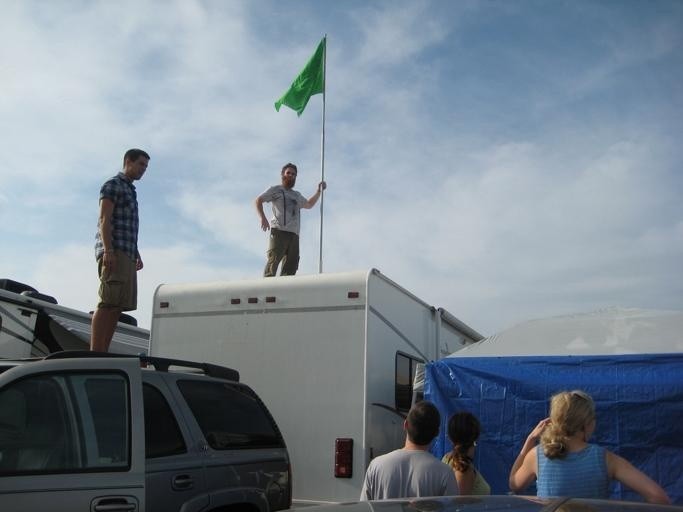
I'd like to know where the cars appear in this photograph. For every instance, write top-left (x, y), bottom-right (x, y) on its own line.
top-left (1, 350), bottom-right (292, 512)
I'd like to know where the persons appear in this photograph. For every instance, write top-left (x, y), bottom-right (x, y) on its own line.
top-left (358, 401), bottom-right (491, 501)
top-left (254, 162), bottom-right (327, 277)
top-left (90, 148), bottom-right (151, 353)
top-left (508, 390), bottom-right (671, 505)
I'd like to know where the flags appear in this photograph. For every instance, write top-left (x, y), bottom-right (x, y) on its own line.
top-left (274, 36), bottom-right (324, 116)
